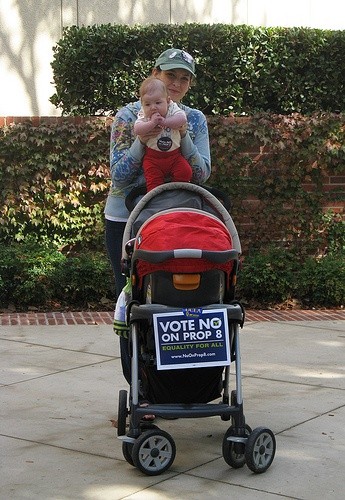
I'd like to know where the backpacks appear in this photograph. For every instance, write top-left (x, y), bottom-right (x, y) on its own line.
top-left (132, 319), bottom-right (222, 420)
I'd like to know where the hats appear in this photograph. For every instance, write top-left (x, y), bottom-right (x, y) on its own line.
top-left (154, 48), bottom-right (197, 78)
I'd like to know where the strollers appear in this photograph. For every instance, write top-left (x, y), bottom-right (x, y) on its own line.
top-left (114, 182), bottom-right (277, 475)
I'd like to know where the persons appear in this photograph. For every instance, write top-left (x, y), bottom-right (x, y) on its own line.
top-left (102, 49), bottom-right (212, 423)
top-left (133, 75), bottom-right (195, 196)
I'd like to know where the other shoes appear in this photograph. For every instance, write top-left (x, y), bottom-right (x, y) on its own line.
top-left (128, 394), bottom-right (156, 426)
